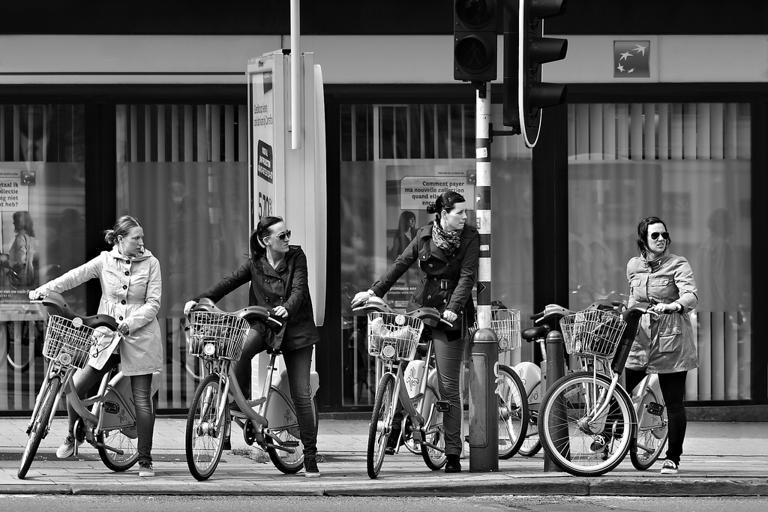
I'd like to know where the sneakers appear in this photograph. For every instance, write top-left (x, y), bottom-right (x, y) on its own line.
top-left (211, 400), bottom-right (249, 419)
top-left (303, 457), bottom-right (321, 478)
top-left (138, 461), bottom-right (155, 477)
top-left (660, 459), bottom-right (678, 474)
top-left (378, 434), bottom-right (404, 448)
top-left (445, 457), bottom-right (461, 473)
top-left (56, 436), bottom-right (84, 459)
top-left (591, 432), bottom-right (616, 454)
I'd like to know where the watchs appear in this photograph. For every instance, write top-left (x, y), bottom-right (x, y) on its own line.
top-left (368, 289), bottom-right (376, 296)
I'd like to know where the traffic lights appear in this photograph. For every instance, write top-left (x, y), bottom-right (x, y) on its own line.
top-left (532, 0), bottom-right (568, 109)
top-left (453, 1), bottom-right (492, 73)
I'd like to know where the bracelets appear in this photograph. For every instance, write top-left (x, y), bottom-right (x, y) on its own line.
top-left (675, 304), bottom-right (682, 313)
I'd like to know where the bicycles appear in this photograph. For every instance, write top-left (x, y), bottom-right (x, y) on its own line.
top-left (349, 294), bottom-right (465, 479)
top-left (0, 258), bottom-right (44, 371)
top-left (178, 298), bottom-right (322, 480)
top-left (10, 292), bottom-right (161, 477)
top-left (473, 297), bottom-right (671, 477)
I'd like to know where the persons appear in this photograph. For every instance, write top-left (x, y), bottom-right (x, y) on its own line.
top-left (184, 216), bottom-right (320, 477)
top-left (391, 211), bottom-right (416, 262)
top-left (29, 215), bottom-right (163, 477)
top-left (350, 191), bottom-right (479, 473)
top-left (589, 217), bottom-right (700, 474)
top-left (9, 211), bottom-right (35, 269)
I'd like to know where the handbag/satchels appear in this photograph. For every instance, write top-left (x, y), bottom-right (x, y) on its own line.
top-left (9, 263), bottom-right (35, 287)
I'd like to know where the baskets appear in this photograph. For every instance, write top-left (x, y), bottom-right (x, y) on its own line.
top-left (558, 311), bottom-right (626, 359)
top-left (188, 310), bottom-right (250, 361)
top-left (42, 314), bottom-right (104, 370)
top-left (469, 309), bottom-right (522, 351)
top-left (366, 312), bottom-right (424, 361)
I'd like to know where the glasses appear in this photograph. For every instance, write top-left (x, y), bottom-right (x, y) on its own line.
top-left (267, 230), bottom-right (291, 241)
top-left (651, 232), bottom-right (669, 239)
